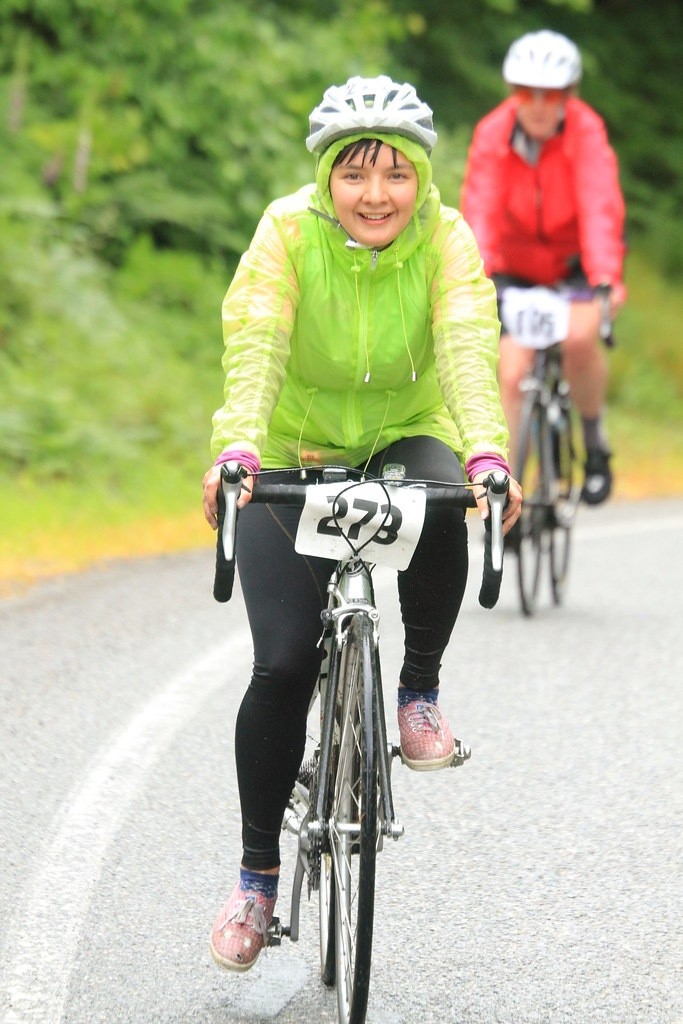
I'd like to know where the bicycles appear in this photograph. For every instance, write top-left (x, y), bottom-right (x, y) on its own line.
top-left (496, 280), bottom-right (621, 615)
top-left (215, 463), bottom-right (512, 1023)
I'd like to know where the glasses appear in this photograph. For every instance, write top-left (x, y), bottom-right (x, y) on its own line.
top-left (511, 83), bottom-right (570, 104)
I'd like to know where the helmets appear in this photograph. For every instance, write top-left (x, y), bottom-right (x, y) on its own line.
top-left (304, 74), bottom-right (439, 157)
top-left (502, 31), bottom-right (584, 89)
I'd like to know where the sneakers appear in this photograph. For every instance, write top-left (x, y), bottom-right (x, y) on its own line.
top-left (397, 700), bottom-right (455, 771)
top-left (210, 881), bottom-right (279, 972)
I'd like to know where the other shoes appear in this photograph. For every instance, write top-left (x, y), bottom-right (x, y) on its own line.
top-left (585, 450), bottom-right (610, 504)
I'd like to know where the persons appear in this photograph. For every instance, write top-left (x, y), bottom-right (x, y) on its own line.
top-left (460, 30), bottom-right (628, 552)
top-left (205, 75), bottom-right (524, 973)
